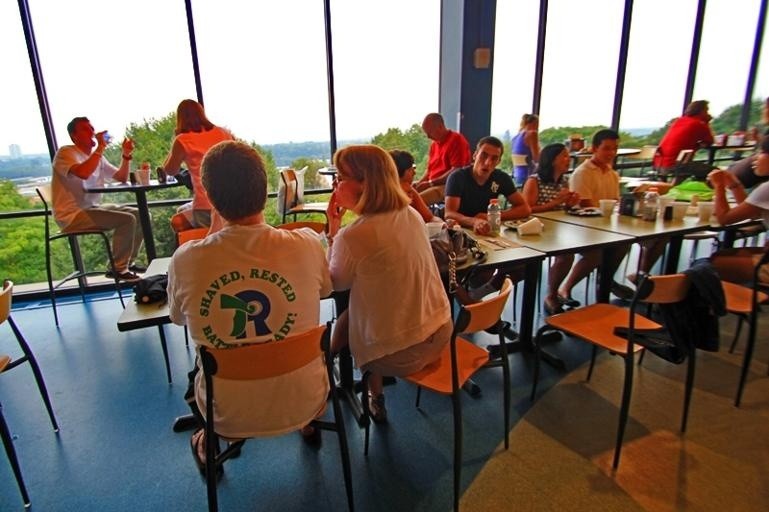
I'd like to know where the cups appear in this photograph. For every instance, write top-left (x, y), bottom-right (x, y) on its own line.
top-left (138, 170), bottom-right (150, 185)
top-left (670, 202), bottom-right (688, 224)
top-left (696, 202), bottom-right (714, 224)
top-left (517, 217), bottom-right (543, 236)
top-left (599, 199), bottom-right (616, 217)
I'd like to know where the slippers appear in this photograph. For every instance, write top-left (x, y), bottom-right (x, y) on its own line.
top-left (303, 422), bottom-right (321, 447)
top-left (191, 427), bottom-right (225, 484)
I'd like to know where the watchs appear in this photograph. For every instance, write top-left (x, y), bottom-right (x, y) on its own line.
top-left (428, 180), bottom-right (434, 188)
top-left (121, 153), bottom-right (133, 161)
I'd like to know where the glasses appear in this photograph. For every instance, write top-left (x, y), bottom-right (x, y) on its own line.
top-left (336, 173), bottom-right (360, 185)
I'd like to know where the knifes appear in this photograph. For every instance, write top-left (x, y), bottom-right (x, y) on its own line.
top-left (485, 239), bottom-right (505, 248)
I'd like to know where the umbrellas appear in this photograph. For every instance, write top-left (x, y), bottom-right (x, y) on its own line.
top-left (130, 276), bottom-right (168, 307)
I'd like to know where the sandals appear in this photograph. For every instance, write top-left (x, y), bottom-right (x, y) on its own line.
top-left (544, 300), bottom-right (565, 315)
top-left (557, 293), bottom-right (580, 307)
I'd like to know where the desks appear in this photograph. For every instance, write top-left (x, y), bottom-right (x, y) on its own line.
top-left (661, 197), bottom-right (769, 254)
top-left (465, 213), bottom-right (637, 371)
top-left (691, 135), bottom-right (759, 171)
top-left (565, 148), bottom-right (642, 180)
top-left (81, 178), bottom-right (188, 281)
top-left (531, 202), bottom-right (711, 314)
top-left (362, 223), bottom-right (547, 422)
top-left (117, 256), bottom-right (367, 436)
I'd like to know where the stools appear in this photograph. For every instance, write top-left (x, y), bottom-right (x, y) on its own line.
top-left (684, 233), bottom-right (720, 263)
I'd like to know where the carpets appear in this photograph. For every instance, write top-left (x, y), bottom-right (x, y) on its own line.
top-left (453, 303), bottom-right (769, 512)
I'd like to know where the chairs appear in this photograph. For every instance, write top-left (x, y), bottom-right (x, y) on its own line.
top-left (528, 262), bottom-right (700, 475)
top-left (623, 244), bottom-right (634, 289)
top-left (279, 168), bottom-right (331, 230)
top-left (729, 278), bottom-right (769, 357)
top-left (198, 320), bottom-right (358, 512)
top-left (274, 221), bottom-right (328, 236)
top-left (536, 256), bottom-right (591, 313)
top-left (624, 148), bottom-right (697, 201)
top-left (34, 183), bottom-right (136, 329)
top-left (735, 226), bottom-right (769, 246)
top-left (361, 276), bottom-right (515, 512)
top-left (0, 278), bottom-right (59, 512)
top-left (638, 252), bottom-right (768, 406)
top-left (156, 227), bottom-right (212, 386)
top-left (659, 233), bottom-right (722, 276)
top-left (511, 280), bottom-right (522, 331)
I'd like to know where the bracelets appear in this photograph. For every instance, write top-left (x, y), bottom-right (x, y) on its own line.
top-left (94, 152), bottom-right (103, 158)
top-left (727, 181), bottom-right (741, 191)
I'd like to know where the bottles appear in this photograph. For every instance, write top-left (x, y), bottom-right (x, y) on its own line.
top-left (619, 192), bottom-right (634, 216)
top-left (92, 133), bottom-right (121, 146)
top-left (641, 187), bottom-right (659, 221)
top-left (487, 198), bottom-right (502, 236)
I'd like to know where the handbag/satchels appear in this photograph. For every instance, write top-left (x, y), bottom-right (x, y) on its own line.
top-left (430, 227), bottom-right (485, 292)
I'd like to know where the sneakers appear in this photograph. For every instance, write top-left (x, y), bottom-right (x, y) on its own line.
top-left (105, 270), bottom-right (139, 283)
top-left (129, 262), bottom-right (148, 273)
top-left (366, 394), bottom-right (388, 423)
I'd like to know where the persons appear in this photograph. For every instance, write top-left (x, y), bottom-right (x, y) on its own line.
top-left (653, 101), bottom-right (713, 187)
top-left (568, 129), bottom-right (668, 299)
top-left (327, 145), bottom-right (454, 426)
top-left (389, 149), bottom-right (444, 225)
top-left (522, 143), bottom-right (598, 315)
top-left (444, 136), bottom-right (532, 334)
top-left (163, 100), bottom-right (236, 233)
top-left (707, 96), bottom-right (769, 191)
top-left (706, 140), bottom-right (769, 286)
top-left (511, 114), bottom-right (541, 185)
top-left (413, 113), bottom-right (472, 207)
top-left (52, 117), bottom-right (153, 279)
top-left (166, 140), bottom-right (335, 484)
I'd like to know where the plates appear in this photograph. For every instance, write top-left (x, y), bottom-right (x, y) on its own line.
top-left (504, 218), bottom-right (544, 228)
